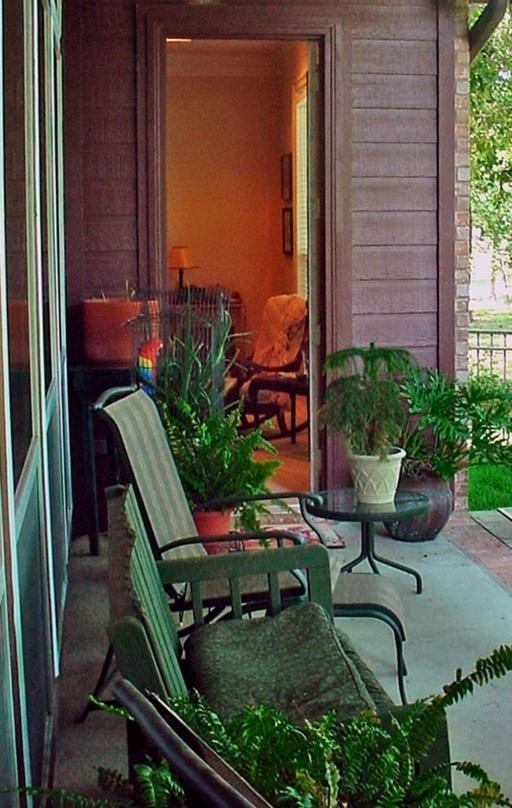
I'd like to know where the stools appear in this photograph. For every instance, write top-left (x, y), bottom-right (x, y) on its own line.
top-left (265, 571), bottom-right (410, 707)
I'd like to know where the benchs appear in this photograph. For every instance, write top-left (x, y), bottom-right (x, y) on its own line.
top-left (101, 482), bottom-right (452, 793)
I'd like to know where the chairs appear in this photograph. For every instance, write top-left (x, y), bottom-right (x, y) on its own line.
top-left (77, 382), bottom-right (341, 724)
top-left (221, 293), bottom-right (310, 445)
top-left (112, 676), bottom-right (274, 808)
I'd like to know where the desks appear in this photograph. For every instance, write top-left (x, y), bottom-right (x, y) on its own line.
top-left (304, 486), bottom-right (432, 595)
top-left (163, 285), bottom-right (247, 369)
top-left (68, 344), bottom-right (143, 556)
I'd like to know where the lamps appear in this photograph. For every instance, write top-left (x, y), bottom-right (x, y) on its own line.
top-left (168, 245), bottom-right (198, 290)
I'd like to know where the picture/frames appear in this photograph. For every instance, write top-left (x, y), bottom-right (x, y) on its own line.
top-left (280, 152), bottom-right (293, 201)
top-left (282, 207), bottom-right (293, 255)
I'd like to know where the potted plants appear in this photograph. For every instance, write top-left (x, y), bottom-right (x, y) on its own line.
top-left (81, 280), bottom-right (160, 365)
top-left (380, 364), bottom-right (512, 542)
top-left (318, 341), bottom-right (417, 506)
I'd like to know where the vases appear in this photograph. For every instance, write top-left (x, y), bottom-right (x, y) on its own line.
top-left (127, 360), bottom-right (301, 556)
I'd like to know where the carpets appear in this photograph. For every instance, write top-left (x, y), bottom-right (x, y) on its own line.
top-left (226, 499), bottom-right (344, 550)
